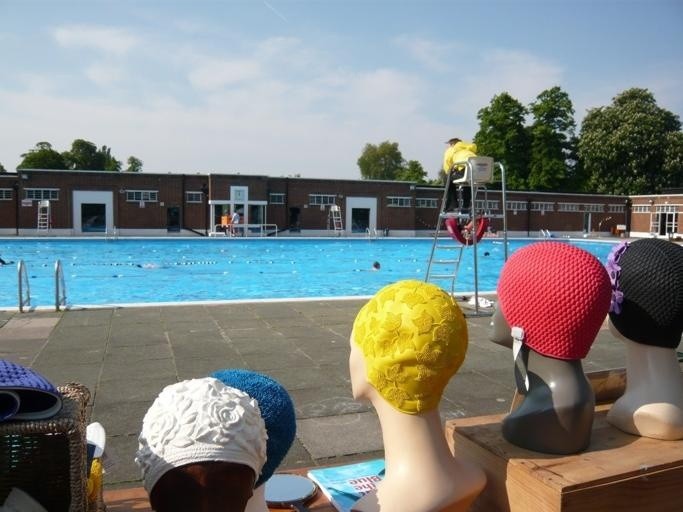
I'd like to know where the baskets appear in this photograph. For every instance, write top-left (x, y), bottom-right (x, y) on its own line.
top-left (0, 380), bottom-right (91, 511)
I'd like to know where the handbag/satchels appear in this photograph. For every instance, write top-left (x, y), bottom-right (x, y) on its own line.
top-left (0, 352), bottom-right (63, 424)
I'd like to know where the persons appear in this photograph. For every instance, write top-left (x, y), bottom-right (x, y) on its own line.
top-left (347, 278), bottom-right (487, 511)
top-left (440, 137), bottom-right (478, 213)
top-left (227, 208), bottom-right (240, 236)
top-left (133, 374), bottom-right (269, 511)
top-left (203, 369), bottom-right (297, 511)
top-left (371, 261), bottom-right (380, 271)
top-left (597, 238), bottom-right (682, 443)
top-left (486, 240), bottom-right (612, 454)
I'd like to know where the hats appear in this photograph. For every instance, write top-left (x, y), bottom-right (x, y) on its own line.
top-left (349, 277), bottom-right (470, 413)
top-left (133, 375), bottom-right (264, 495)
top-left (606, 236), bottom-right (683, 349)
top-left (443, 137), bottom-right (462, 144)
top-left (494, 239), bottom-right (613, 362)
top-left (201, 368), bottom-right (297, 488)
